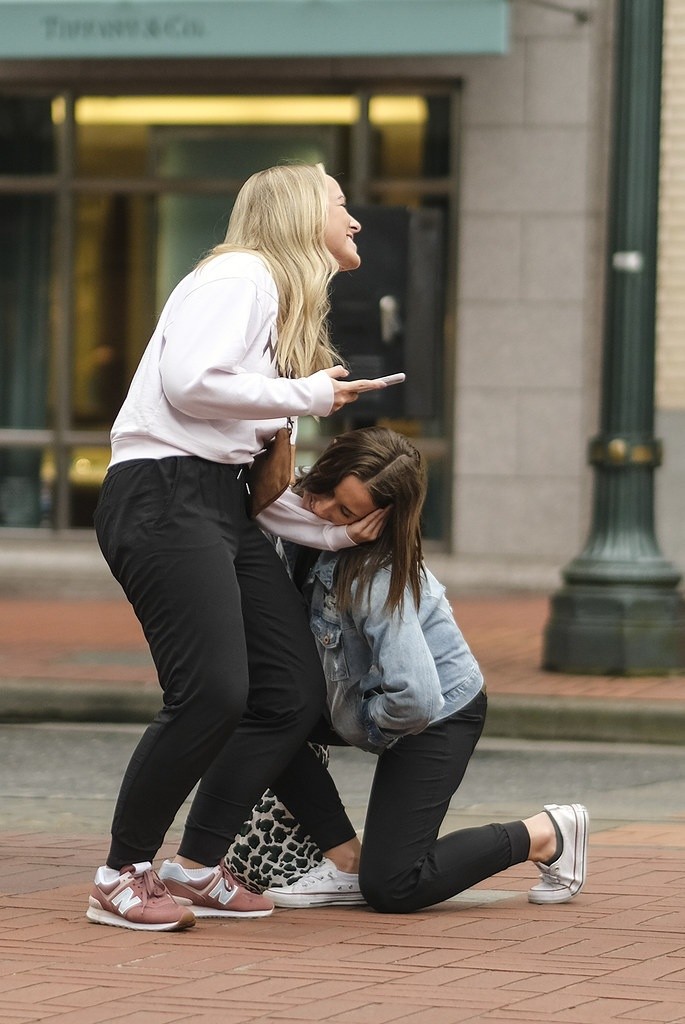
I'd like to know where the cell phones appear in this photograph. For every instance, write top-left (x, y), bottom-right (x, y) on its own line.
top-left (355, 373), bottom-right (406, 392)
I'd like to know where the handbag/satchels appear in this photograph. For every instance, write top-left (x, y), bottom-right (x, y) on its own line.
top-left (244, 415), bottom-right (296, 520)
top-left (224, 742), bottom-right (330, 895)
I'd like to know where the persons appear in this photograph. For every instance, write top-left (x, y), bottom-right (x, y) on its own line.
top-left (85, 164), bottom-right (386, 932)
top-left (262, 426), bottom-right (588, 917)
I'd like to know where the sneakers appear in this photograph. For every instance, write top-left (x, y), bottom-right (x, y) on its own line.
top-left (157, 859), bottom-right (274, 918)
top-left (527, 803), bottom-right (589, 904)
top-left (262, 858), bottom-right (369, 909)
top-left (85, 862), bottom-right (196, 931)
